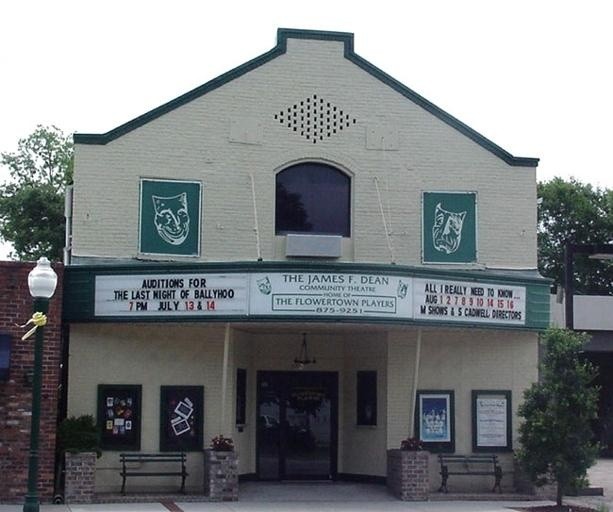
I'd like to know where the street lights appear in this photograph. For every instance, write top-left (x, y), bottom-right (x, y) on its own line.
top-left (563, 243), bottom-right (613, 335)
top-left (19, 255), bottom-right (59, 509)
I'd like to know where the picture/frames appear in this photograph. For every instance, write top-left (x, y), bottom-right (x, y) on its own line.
top-left (471, 389), bottom-right (512, 452)
top-left (415, 388), bottom-right (455, 453)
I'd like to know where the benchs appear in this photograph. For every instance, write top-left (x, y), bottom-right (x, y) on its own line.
top-left (120, 453), bottom-right (189, 495)
top-left (438, 452), bottom-right (503, 494)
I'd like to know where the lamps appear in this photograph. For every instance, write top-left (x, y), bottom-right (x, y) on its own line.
top-left (294, 332), bottom-right (316, 367)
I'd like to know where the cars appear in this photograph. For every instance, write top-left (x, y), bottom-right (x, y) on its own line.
top-left (258, 414), bottom-right (279, 428)
